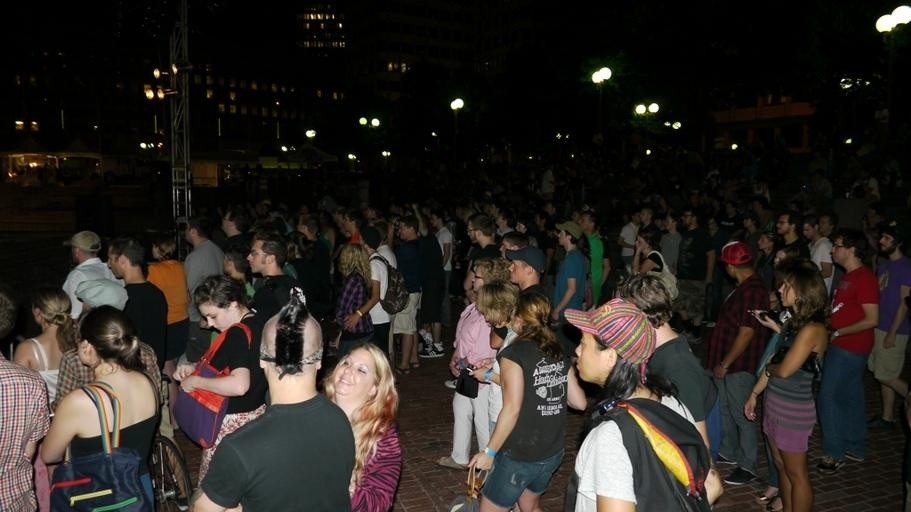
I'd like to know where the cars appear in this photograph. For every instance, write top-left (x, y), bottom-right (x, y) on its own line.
top-left (221, 162), bottom-right (346, 188)
top-left (56, 155), bottom-right (194, 190)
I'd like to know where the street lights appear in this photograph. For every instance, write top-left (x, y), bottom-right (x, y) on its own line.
top-left (275, 65), bottom-right (664, 176)
top-left (873, 2), bottom-right (911, 153)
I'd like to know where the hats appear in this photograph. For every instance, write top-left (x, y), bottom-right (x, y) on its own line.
top-left (555, 222), bottom-right (583, 240)
top-left (721, 241), bottom-right (751, 265)
top-left (564, 298), bottom-right (656, 364)
top-left (505, 246), bottom-right (546, 274)
top-left (878, 219), bottom-right (903, 238)
top-left (63, 231), bottom-right (101, 251)
top-left (75, 279), bottom-right (128, 311)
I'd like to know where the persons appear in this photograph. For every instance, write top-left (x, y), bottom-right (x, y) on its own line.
top-left (2, 160), bottom-right (910, 511)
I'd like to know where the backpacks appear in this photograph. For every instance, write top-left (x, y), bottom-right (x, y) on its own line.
top-left (369, 256), bottom-right (408, 314)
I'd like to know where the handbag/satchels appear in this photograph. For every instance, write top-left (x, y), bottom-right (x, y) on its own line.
top-left (771, 347), bottom-right (818, 372)
top-left (454, 369), bottom-right (478, 399)
top-left (173, 323), bottom-right (252, 449)
top-left (50, 382), bottom-right (154, 512)
top-left (448, 462), bottom-right (489, 512)
top-left (646, 251), bottom-right (679, 300)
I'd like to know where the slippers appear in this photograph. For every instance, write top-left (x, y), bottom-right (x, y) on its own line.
top-left (436, 456), bottom-right (468, 469)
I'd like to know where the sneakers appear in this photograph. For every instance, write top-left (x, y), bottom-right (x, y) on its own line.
top-left (816, 455), bottom-right (846, 475)
top-left (714, 452), bottom-right (756, 485)
top-left (416, 329), bottom-right (445, 358)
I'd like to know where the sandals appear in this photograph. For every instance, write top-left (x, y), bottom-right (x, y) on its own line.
top-left (754, 494), bottom-right (784, 512)
top-left (394, 362), bottom-right (419, 375)
